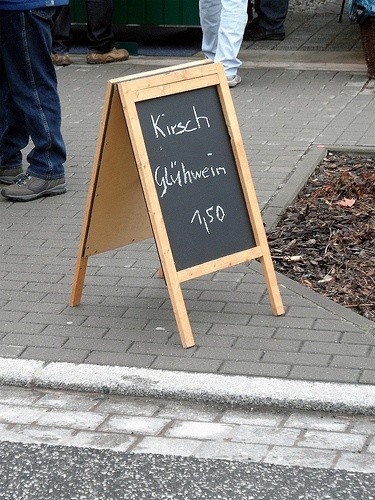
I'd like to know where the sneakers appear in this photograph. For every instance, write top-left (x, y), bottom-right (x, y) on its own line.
top-left (0, 174), bottom-right (68, 202)
top-left (0, 165), bottom-right (24, 184)
top-left (51, 51), bottom-right (70, 66)
top-left (85, 47), bottom-right (130, 64)
top-left (224, 73), bottom-right (241, 87)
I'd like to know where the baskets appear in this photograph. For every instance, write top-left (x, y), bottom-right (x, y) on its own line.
top-left (359, 15), bottom-right (375, 78)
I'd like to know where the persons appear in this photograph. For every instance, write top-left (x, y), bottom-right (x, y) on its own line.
top-left (50, 0), bottom-right (129, 66)
top-left (243, 0), bottom-right (289, 41)
top-left (0, 0), bottom-right (70, 201)
top-left (196, 0), bottom-right (248, 87)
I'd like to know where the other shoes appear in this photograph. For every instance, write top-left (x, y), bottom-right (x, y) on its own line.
top-left (244, 24), bottom-right (286, 40)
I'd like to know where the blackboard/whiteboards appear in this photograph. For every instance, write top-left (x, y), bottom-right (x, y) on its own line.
top-left (71, 60), bottom-right (270, 285)
